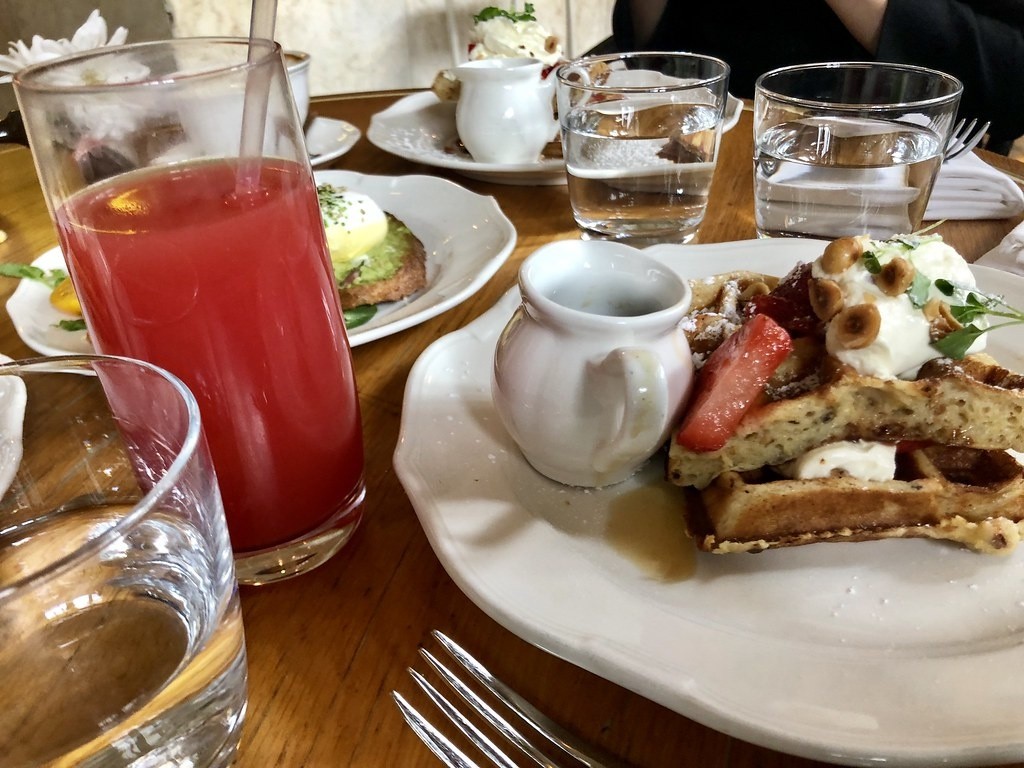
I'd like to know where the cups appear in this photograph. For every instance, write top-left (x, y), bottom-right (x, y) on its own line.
top-left (494, 240), bottom-right (695, 490)
top-left (752, 61), bottom-right (965, 240)
top-left (0, 351), bottom-right (250, 768)
top-left (164, 48), bottom-right (311, 158)
top-left (453, 56), bottom-right (595, 167)
top-left (555, 51), bottom-right (730, 250)
top-left (14, 36), bottom-right (373, 589)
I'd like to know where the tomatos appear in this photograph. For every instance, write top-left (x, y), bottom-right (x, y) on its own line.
top-left (675, 316), bottom-right (793, 451)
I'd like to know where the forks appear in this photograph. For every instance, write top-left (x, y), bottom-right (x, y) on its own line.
top-left (942, 118), bottom-right (992, 163)
top-left (389, 630), bottom-right (638, 768)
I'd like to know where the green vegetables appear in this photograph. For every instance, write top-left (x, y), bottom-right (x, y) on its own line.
top-left (858, 217), bottom-right (1024, 360)
top-left (472, 2), bottom-right (536, 23)
top-left (314, 181), bottom-right (366, 230)
top-left (0, 261), bottom-right (87, 330)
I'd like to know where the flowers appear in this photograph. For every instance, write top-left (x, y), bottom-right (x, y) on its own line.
top-left (0, 8), bottom-right (151, 144)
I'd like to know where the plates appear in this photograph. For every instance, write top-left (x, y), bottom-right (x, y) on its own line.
top-left (392, 237), bottom-right (1024, 768)
top-left (0, 355), bottom-right (27, 503)
top-left (4, 170), bottom-right (517, 364)
top-left (148, 116), bottom-right (361, 168)
top-left (366, 70), bottom-right (743, 187)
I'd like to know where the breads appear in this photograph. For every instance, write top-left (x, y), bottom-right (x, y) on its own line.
top-left (329, 211), bottom-right (429, 310)
top-left (674, 268), bottom-right (1023, 553)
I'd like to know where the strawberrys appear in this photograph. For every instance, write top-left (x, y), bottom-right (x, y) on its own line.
top-left (744, 261), bottom-right (820, 334)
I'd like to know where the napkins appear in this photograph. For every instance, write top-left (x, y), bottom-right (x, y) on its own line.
top-left (754, 112), bottom-right (1024, 278)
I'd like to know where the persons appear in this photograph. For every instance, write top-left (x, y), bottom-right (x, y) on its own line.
top-left (611, 0), bottom-right (1024, 156)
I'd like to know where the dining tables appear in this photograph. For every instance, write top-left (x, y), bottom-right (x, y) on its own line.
top-left (0, 92), bottom-right (1024, 767)
